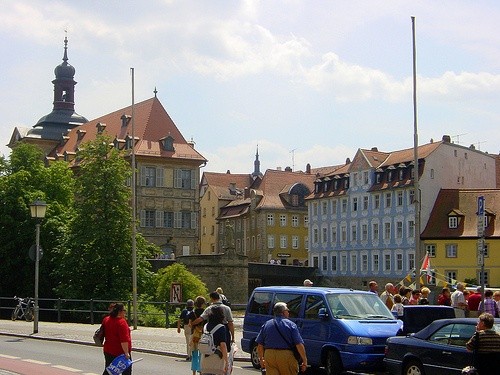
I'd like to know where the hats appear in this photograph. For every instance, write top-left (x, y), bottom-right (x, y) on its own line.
top-left (304, 279), bottom-right (313, 285)
top-left (463, 290), bottom-right (469, 295)
top-left (215, 287), bottom-right (223, 294)
top-left (186, 300), bottom-right (194, 303)
top-left (421, 288), bottom-right (430, 294)
top-left (477, 286), bottom-right (481, 291)
top-left (493, 291), bottom-right (500, 296)
top-left (396, 284), bottom-right (402, 286)
top-left (404, 288), bottom-right (412, 292)
top-left (456, 282), bottom-right (466, 289)
top-left (197, 298), bottom-right (204, 304)
top-left (412, 290), bottom-right (419, 294)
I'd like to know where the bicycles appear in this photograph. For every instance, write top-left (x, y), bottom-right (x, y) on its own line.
top-left (11, 296), bottom-right (36, 322)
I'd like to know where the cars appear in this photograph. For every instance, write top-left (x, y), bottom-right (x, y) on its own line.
top-left (383, 304), bottom-right (500, 375)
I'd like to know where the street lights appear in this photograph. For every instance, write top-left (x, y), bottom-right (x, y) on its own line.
top-left (27, 198), bottom-right (50, 334)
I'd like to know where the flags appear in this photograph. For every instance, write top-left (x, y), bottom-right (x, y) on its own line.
top-left (422, 255), bottom-right (433, 283)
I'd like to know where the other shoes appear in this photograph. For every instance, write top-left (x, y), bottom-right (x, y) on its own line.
top-left (186, 357), bottom-right (191, 361)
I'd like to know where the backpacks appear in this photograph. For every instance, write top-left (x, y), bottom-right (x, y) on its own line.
top-left (198, 323), bottom-right (226, 355)
top-left (220, 295), bottom-right (232, 310)
top-left (191, 326), bottom-right (204, 350)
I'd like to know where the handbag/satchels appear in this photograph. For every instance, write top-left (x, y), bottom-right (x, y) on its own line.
top-left (105, 354), bottom-right (132, 375)
top-left (461, 365), bottom-right (478, 375)
top-left (93, 324), bottom-right (105, 346)
top-left (292, 345), bottom-right (303, 365)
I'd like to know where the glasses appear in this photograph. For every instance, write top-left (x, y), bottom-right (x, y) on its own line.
top-left (121, 310), bottom-right (126, 312)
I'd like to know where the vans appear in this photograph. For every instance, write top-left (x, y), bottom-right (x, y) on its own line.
top-left (241, 285), bottom-right (404, 375)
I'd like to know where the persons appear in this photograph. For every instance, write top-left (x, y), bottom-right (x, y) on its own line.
top-left (437, 288), bottom-right (451, 307)
top-left (462, 285), bottom-right (500, 318)
top-left (419, 286), bottom-right (431, 305)
top-left (379, 282), bottom-right (395, 310)
top-left (391, 294), bottom-right (404, 315)
top-left (450, 283), bottom-right (467, 308)
top-left (190, 291), bottom-right (237, 375)
top-left (187, 296), bottom-right (206, 375)
top-left (408, 289), bottom-right (421, 305)
top-left (176, 299), bottom-right (195, 361)
top-left (216, 287), bottom-right (228, 302)
top-left (255, 301), bottom-right (307, 375)
top-left (464, 312), bottom-right (500, 375)
top-left (201, 306), bottom-right (230, 375)
top-left (400, 287), bottom-right (412, 306)
top-left (269, 258), bottom-right (281, 265)
top-left (97, 303), bottom-right (133, 375)
top-left (304, 280), bottom-right (318, 304)
top-left (367, 280), bottom-right (379, 305)
